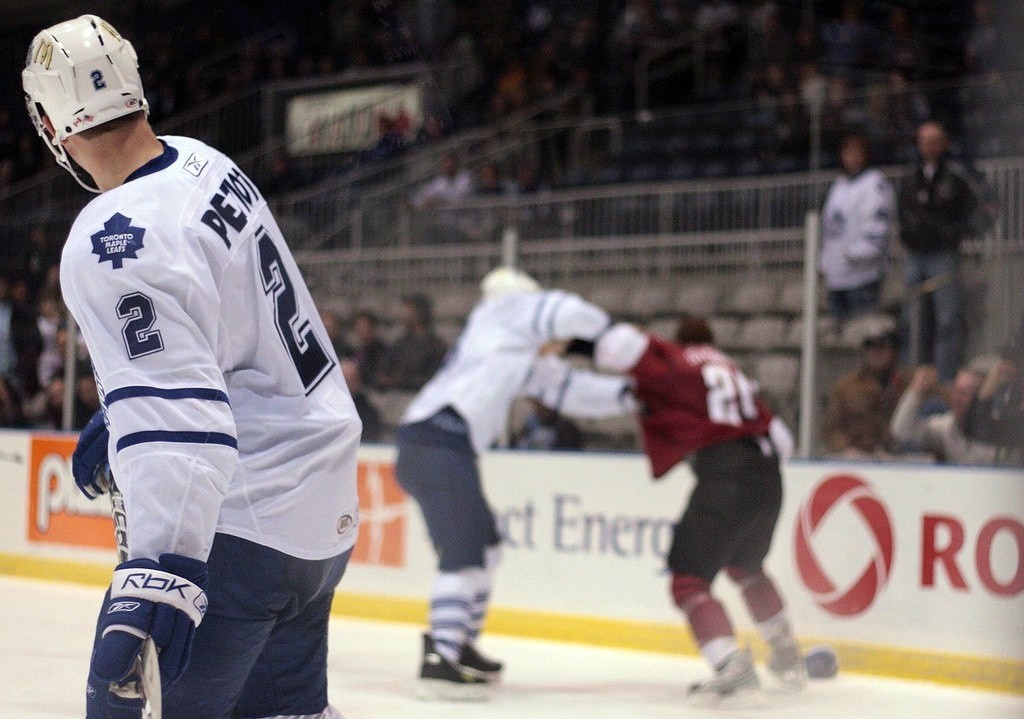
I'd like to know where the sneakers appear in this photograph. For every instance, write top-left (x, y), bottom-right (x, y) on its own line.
top-left (458, 643), bottom-right (504, 681)
top-left (688, 639), bottom-right (761, 700)
top-left (418, 634), bottom-right (491, 700)
top-left (767, 621), bottom-right (810, 691)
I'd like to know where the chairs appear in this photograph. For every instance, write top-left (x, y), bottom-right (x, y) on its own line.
top-left (323, 272), bottom-right (914, 451)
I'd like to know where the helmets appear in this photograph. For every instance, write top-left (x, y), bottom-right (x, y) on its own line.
top-left (22, 14), bottom-right (150, 140)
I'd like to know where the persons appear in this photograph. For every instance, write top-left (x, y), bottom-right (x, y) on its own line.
top-left (0, 0), bottom-right (1024, 698)
top-left (23, 15), bottom-right (362, 719)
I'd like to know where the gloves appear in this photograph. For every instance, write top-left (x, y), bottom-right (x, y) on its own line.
top-left (72, 411), bottom-right (113, 500)
top-left (91, 554), bottom-right (210, 709)
